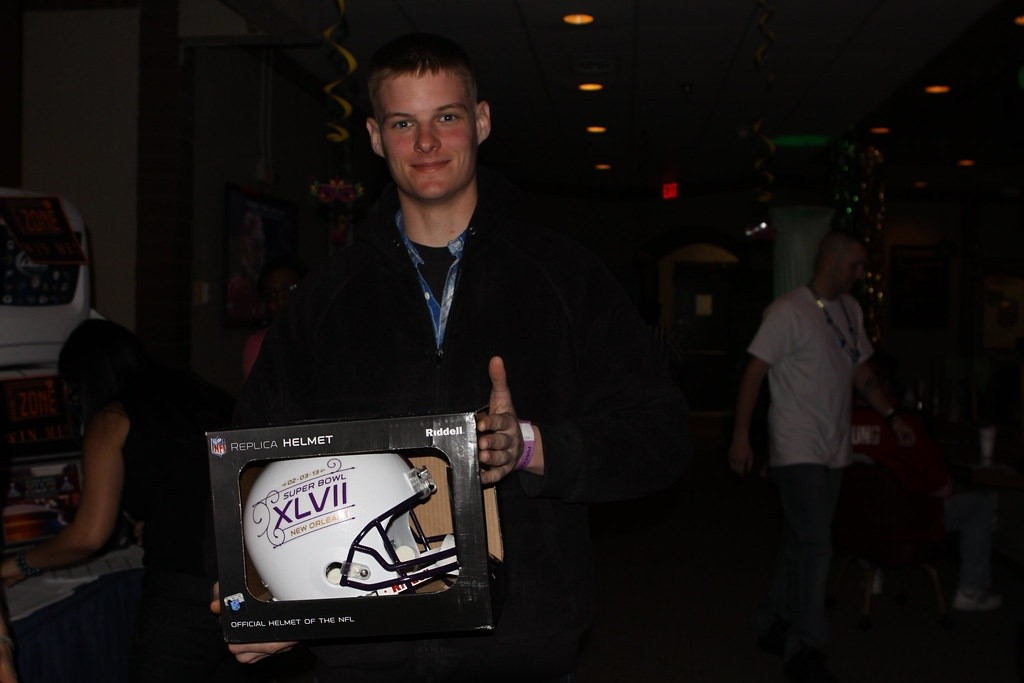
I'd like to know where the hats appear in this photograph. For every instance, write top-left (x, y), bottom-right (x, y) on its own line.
top-left (258, 254), bottom-right (309, 292)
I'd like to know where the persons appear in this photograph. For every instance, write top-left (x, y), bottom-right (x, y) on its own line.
top-left (209, 33), bottom-right (693, 683)
top-left (0, 255), bottom-right (307, 683)
top-left (729, 225), bottom-right (919, 683)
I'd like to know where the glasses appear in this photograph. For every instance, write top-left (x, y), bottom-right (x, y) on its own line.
top-left (263, 282), bottom-right (298, 301)
top-left (67, 384), bottom-right (80, 406)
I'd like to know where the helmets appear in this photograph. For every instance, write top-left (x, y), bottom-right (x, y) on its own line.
top-left (244, 452), bottom-right (460, 601)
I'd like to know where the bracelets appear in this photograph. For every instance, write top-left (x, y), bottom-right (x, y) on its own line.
top-left (885, 410), bottom-right (899, 423)
top-left (515, 418), bottom-right (537, 472)
top-left (14, 550), bottom-right (42, 576)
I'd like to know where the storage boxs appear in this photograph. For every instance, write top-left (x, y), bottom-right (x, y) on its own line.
top-left (204, 402), bottom-right (509, 645)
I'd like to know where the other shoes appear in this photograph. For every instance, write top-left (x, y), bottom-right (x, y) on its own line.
top-left (784, 642), bottom-right (838, 683)
top-left (758, 619), bottom-right (792, 656)
top-left (951, 590), bottom-right (1002, 618)
